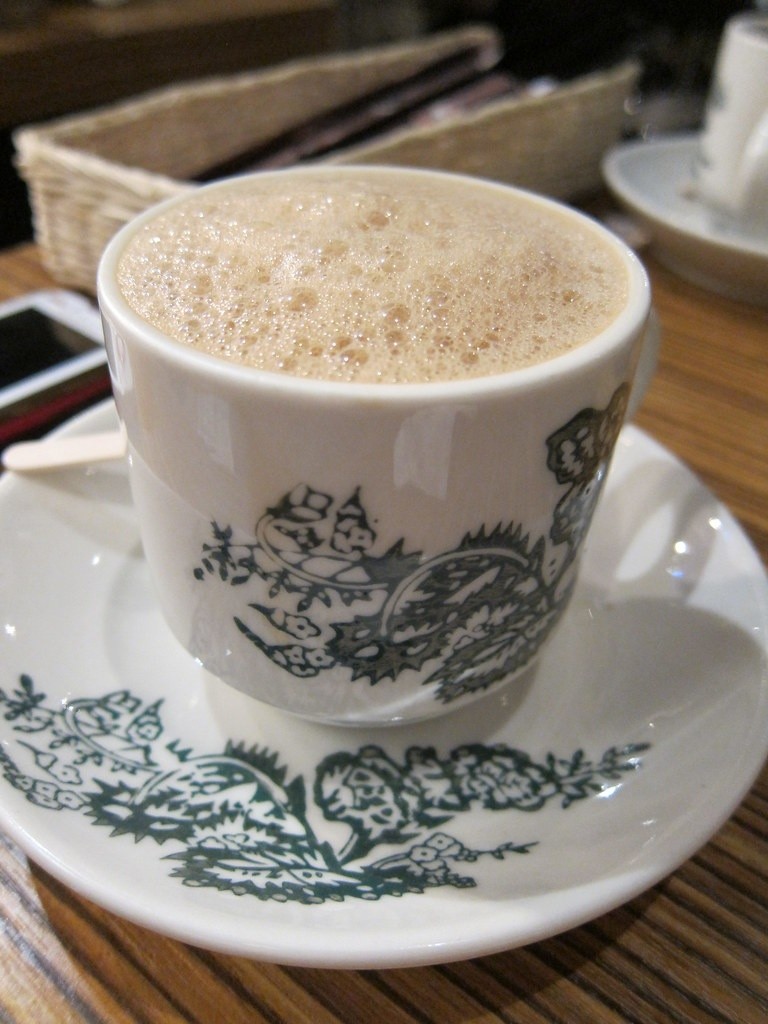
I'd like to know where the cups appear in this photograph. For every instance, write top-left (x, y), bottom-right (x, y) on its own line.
top-left (692, 13), bottom-right (768, 214)
top-left (97, 165), bottom-right (652, 728)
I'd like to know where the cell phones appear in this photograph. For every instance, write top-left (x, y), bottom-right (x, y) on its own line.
top-left (0, 287), bottom-right (110, 422)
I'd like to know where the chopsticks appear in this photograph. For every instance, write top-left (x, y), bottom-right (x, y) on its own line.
top-left (187, 42), bottom-right (507, 184)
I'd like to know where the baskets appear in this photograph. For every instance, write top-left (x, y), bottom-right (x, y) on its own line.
top-left (11, 27), bottom-right (645, 305)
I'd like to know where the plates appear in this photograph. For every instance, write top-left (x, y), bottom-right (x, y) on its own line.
top-left (0, 396), bottom-right (768, 969)
top-left (599, 135), bottom-right (768, 305)
